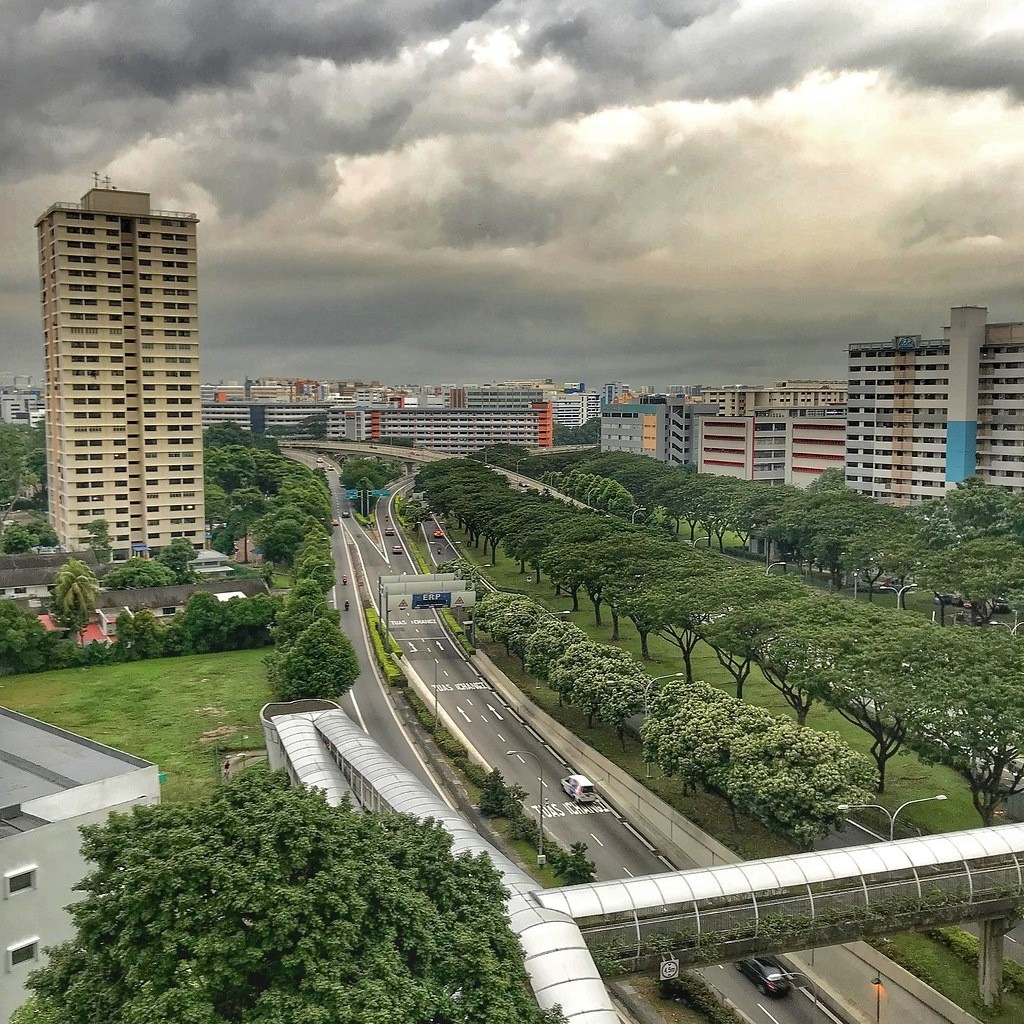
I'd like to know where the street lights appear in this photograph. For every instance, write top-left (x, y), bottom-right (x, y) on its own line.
top-left (692, 536), bottom-right (709, 548)
top-left (409, 649), bottom-right (439, 732)
top-left (506, 749), bottom-right (546, 870)
top-left (429, 539), bottom-right (461, 562)
top-left (516, 458), bottom-right (527, 483)
top-left (605, 672), bottom-right (684, 778)
top-left (311, 599), bottom-right (334, 625)
top-left (836, 794), bottom-right (950, 841)
top-left (587, 487), bottom-right (600, 507)
top-left (452, 563), bottom-right (492, 591)
top-left (504, 609), bottom-right (571, 690)
top-left (485, 447), bottom-right (494, 464)
top-left (550, 472), bottom-right (563, 494)
top-left (766, 562), bottom-right (787, 575)
top-left (310, 563), bottom-right (330, 580)
top-left (879, 582), bottom-right (918, 612)
top-left (632, 507), bottom-right (646, 525)
top-left (768, 972), bottom-right (817, 1024)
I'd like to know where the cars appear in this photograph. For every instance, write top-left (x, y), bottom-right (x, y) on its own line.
top-left (734, 955), bottom-right (792, 999)
top-left (560, 774), bottom-right (598, 804)
top-left (316, 443), bottom-right (443, 554)
top-left (518, 481), bottom-right (529, 487)
top-left (932, 589), bottom-right (1013, 615)
